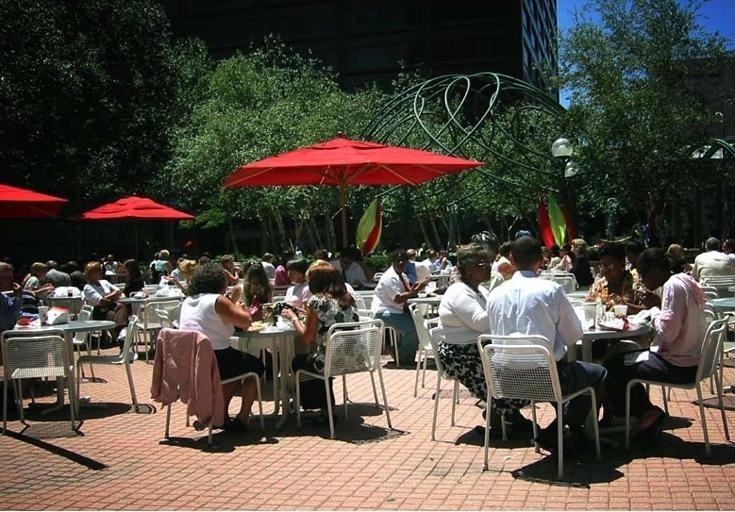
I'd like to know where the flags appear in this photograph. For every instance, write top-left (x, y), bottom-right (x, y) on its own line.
top-left (355, 196), bottom-right (383, 257)
top-left (538, 194), bottom-right (574, 249)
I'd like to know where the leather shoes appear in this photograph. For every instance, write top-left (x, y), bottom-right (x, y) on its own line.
top-left (533, 427), bottom-right (572, 460)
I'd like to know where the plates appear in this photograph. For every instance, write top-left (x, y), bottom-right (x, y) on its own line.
top-left (248, 325), bottom-right (264, 332)
top-left (599, 321), bottom-right (642, 331)
top-left (14, 324), bottom-right (30, 328)
top-left (417, 293), bottom-right (428, 297)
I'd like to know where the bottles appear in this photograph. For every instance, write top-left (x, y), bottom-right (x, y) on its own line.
top-left (571, 301), bottom-right (613, 330)
top-left (263, 303), bottom-right (282, 325)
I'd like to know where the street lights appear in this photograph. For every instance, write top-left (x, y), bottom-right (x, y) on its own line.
top-left (550, 136), bottom-right (580, 245)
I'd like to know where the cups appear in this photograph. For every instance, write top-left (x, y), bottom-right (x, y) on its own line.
top-left (425, 281), bottom-right (436, 296)
top-left (614, 304), bottom-right (628, 318)
top-left (141, 288), bottom-right (149, 297)
top-left (38, 306), bottom-right (48, 326)
top-left (67, 289), bottom-right (73, 297)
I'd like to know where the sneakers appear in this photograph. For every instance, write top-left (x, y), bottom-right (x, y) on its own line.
top-left (629, 405), bottom-right (667, 447)
top-left (482, 406), bottom-right (541, 440)
top-left (598, 414), bottom-right (638, 435)
top-left (210, 413), bottom-right (250, 433)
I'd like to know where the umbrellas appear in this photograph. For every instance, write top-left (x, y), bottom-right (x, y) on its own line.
top-left (221, 131), bottom-right (488, 250)
top-left (72, 191), bottom-right (196, 259)
top-left (0, 183), bottom-right (68, 222)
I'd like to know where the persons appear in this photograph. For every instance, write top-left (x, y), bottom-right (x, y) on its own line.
top-left (372, 242), bottom-right (442, 369)
top-left (1, 255), bottom-right (144, 347)
top-left (437, 238), bottom-right (734, 461)
top-left (150, 250), bottom-right (368, 432)
